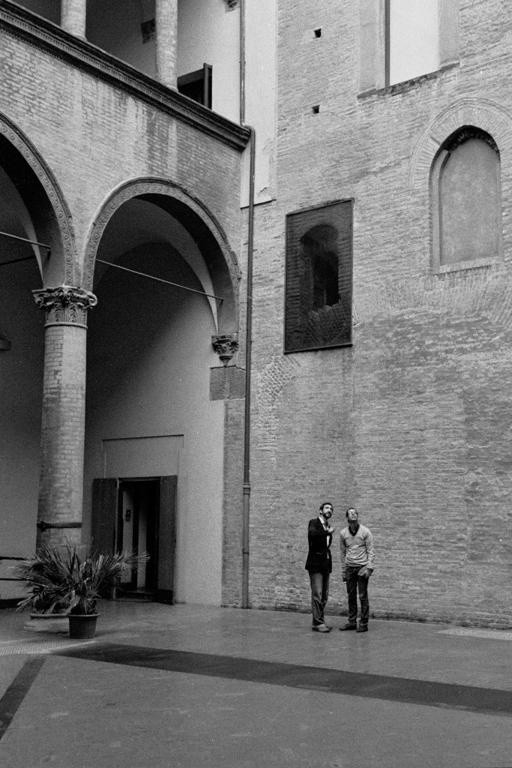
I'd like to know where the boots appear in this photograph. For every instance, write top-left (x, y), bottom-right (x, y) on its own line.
top-left (339, 622), bottom-right (356, 631)
top-left (356, 623), bottom-right (370, 632)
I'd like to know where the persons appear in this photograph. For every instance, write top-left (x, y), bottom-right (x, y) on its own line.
top-left (305, 503), bottom-right (335, 633)
top-left (338, 507), bottom-right (376, 631)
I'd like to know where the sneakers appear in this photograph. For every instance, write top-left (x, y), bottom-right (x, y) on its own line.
top-left (312, 624), bottom-right (333, 632)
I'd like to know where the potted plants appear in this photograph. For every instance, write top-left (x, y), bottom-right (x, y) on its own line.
top-left (9, 537), bottom-right (150, 639)
